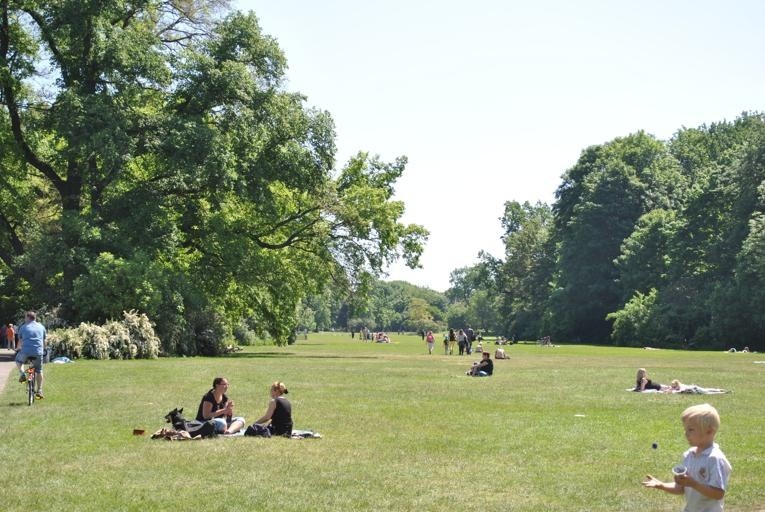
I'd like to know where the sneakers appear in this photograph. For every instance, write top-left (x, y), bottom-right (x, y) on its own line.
top-left (19, 377), bottom-right (26, 382)
top-left (36, 392), bottom-right (45, 399)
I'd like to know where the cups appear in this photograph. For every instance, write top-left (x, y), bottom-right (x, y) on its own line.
top-left (673, 466), bottom-right (687, 491)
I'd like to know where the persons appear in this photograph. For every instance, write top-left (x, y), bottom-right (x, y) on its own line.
top-left (633, 368), bottom-right (672, 392)
top-left (465, 352), bottom-right (493, 377)
top-left (426, 330), bottom-right (436, 355)
top-left (456, 328), bottom-right (468, 355)
top-left (665, 379), bottom-right (730, 394)
top-left (351, 329), bottom-right (355, 338)
top-left (421, 329), bottom-right (425, 340)
top-left (303, 328), bottom-right (307, 340)
top-left (495, 336), bottom-right (501, 345)
top-left (501, 335), bottom-right (508, 345)
top-left (7, 323), bottom-right (16, 351)
top-left (197, 377), bottom-right (245, 436)
top-left (359, 326), bottom-right (390, 344)
top-left (639, 403), bottom-right (733, 512)
top-left (255, 382), bottom-right (293, 435)
top-left (443, 333), bottom-right (449, 355)
top-left (476, 342), bottom-right (484, 352)
top-left (15, 310), bottom-right (46, 399)
top-left (13, 324), bottom-right (20, 345)
top-left (466, 337), bottom-right (473, 354)
top-left (1, 323), bottom-right (9, 348)
top-left (448, 328), bottom-right (457, 355)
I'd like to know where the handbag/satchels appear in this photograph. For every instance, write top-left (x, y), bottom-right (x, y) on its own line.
top-left (244, 423), bottom-right (271, 438)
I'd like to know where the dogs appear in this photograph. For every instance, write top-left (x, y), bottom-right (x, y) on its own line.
top-left (163, 405), bottom-right (192, 432)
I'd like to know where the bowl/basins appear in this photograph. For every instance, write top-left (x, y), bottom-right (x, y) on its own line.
top-left (133, 430), bottom-right (145, 435)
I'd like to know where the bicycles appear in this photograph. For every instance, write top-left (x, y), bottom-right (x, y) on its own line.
top-left (21, 356), bottom-right (41, 406)
